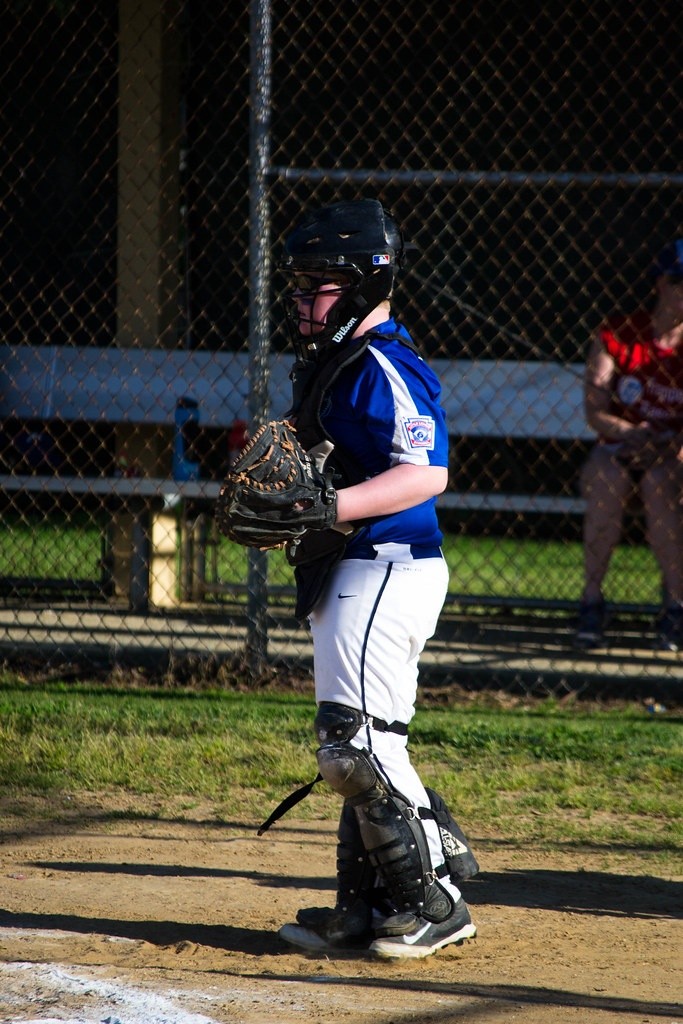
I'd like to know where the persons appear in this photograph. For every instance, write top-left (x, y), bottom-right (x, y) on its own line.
top-left (573, 241), bottom-right (683, 650)
top-left (215, 197), bottom-right (477, 958)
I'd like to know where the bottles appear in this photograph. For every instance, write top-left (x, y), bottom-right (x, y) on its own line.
top-left (228, 419), bottom-right (249, 466)
top-left (175, 395), bottom-right (202, 480)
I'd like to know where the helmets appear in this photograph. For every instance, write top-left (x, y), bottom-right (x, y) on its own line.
top-left (285, 199), bottom-right (404, 368)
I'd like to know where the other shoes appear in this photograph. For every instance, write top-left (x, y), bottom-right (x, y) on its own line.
top-left (574, 595), bottom-right (608, 649)
top-left (654, 605), bottom-right (683, 650)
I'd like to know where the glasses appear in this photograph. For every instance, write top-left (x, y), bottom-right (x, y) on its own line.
top-left (665, 272), bottom-right (683, 285)
top-left (285, 274), bottom-right (345, 293)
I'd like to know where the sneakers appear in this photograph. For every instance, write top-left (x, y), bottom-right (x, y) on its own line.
top-left (368, 894), bottom-right (476, 961)
top-left (274, 911), bottom-right (383, 955)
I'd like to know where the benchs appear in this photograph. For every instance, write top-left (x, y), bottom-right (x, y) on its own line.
top-left (0, 346), bottom-right (603, 513)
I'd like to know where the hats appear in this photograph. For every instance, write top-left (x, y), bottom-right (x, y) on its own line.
top-left (658, 240), bottom-right (683, 277)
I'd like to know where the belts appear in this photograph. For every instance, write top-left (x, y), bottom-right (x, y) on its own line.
top-left (340, 539), bottom-right (444, 559)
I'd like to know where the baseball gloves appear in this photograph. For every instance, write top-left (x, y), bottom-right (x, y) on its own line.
top-left (212, 418), bottom-right (338, 552)
